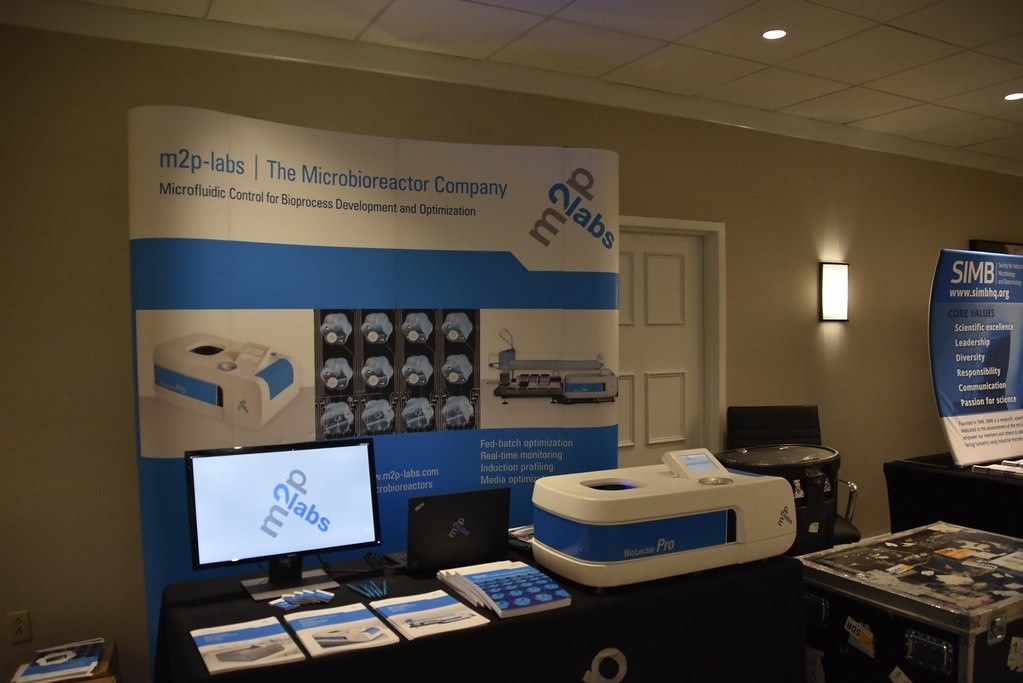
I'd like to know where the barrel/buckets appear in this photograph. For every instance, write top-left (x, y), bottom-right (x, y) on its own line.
top-left (714, 443), bottom-right (841, 557)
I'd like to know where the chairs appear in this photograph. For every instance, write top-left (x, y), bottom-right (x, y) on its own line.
top-left (727, 405), bottom-right (861, 549)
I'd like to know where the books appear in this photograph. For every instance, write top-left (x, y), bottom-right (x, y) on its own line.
top-left (11, 637), bottom-right (103, 683)
top-left (368, 588), bottom-right (491, 641)
top-left (190, 616), bottom-right (305, 673)
top-left (438, 559), bottom-right (572, 617)
top-left (284, 602), bottom-right (400, 657)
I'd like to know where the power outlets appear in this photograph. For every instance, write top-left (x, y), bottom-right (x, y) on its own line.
top-left (7, 610), bottom-right (32, 646)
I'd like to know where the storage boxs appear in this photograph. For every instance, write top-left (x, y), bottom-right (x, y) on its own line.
top-left (793, 521), bottom-right (1023, 683)
top-left (715, 444), bottom-right (842, 546)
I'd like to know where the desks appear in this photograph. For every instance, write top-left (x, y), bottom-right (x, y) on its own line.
top-left (884, 452), bottom-right (1023, 538)
top-left (153, 528), bottom-right (795, 683)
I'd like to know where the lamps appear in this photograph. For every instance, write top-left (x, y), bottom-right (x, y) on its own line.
top-left (818, 262), bottom-right (849, 322)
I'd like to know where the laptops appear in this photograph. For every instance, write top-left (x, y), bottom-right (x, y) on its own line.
top-left (383, 487), bottom-right (511, 577)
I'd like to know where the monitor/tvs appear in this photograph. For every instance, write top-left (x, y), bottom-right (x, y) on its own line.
top-left (184, 437), bottom-right (381, 601)
